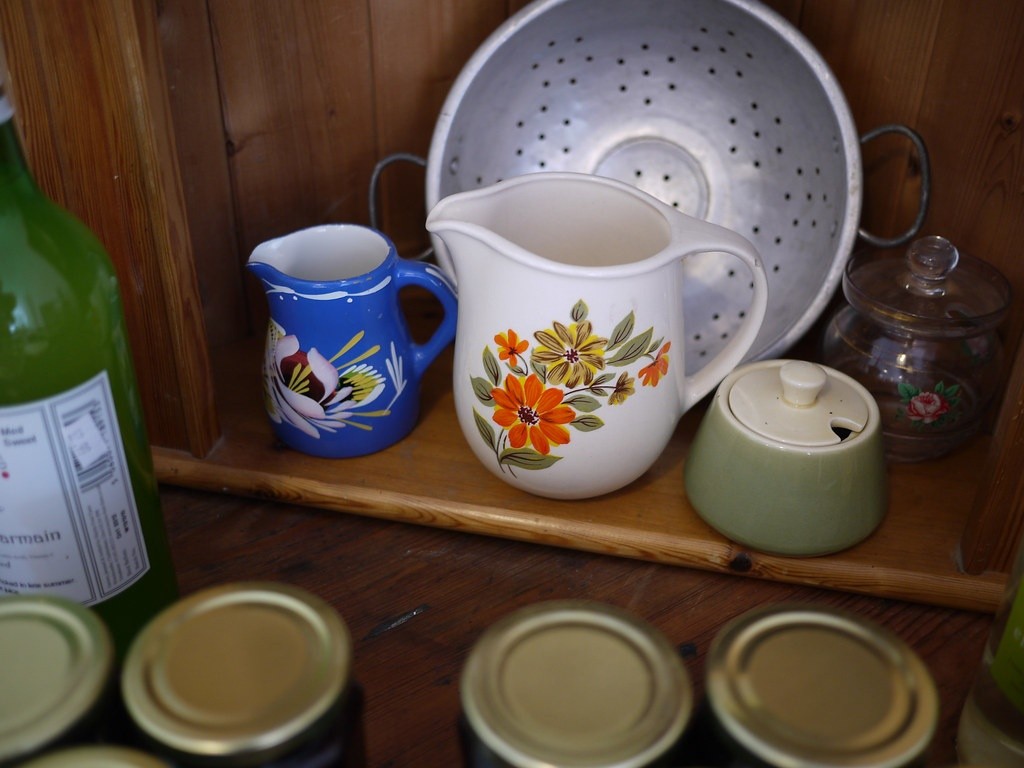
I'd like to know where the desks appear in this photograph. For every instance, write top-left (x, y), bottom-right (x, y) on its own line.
top-left (2, 482), bottom-right (1024, 768)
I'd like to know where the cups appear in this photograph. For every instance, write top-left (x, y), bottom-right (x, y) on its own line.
top-left (684, 359), bottom-right (889, 557)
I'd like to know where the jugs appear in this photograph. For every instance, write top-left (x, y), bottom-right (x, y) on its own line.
top-left (245, 223), bottom-right (457, 457)
top-left (425, 171), bottom-right (770, 498)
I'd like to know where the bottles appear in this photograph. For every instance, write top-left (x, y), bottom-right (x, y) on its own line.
top-left (952, 540), bottom-right (1024, 767)
top-left (1, 72), bottom-right (175, 675)
top-left (814, 236), bottom-right (1012, 461)
